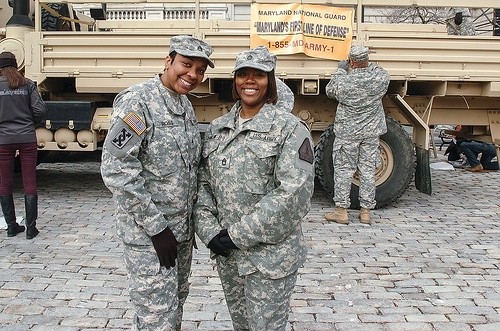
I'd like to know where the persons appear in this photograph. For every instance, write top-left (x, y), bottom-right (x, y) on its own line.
top-left (100, 34), bottom-right (215, 331)
top-left (446, 124), bottom-right (500, 172)
top-left (0, 52), bottom-right (47, 241)
top-left (447, 7), bottom-right (474, 36)
top-left (324, 44), bottom-right (390, 223)
top-left (253, 46), bottom-right (295, 113)
top-left (193, 48), bottom-right (314, 331)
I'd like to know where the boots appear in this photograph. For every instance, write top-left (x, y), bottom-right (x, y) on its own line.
top-left (24, 194), bottom-right (40, 239)
top-left (0, 196), bottom-right (25, 237)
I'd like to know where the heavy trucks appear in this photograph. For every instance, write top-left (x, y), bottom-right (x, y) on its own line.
top-left (0, 0), bottom-right (500, 210)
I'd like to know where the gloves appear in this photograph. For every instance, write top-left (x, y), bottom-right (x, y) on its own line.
top-left (338, 61), bottom-right (350, 71)
top-left (208, 234), bottom-right (230, 257)
top-left (150, 226), bottom-right (179, 269)
top-left (219, 230), bottom-right (240, 249)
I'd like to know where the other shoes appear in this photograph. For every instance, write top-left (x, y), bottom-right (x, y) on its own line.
top-left (324, 208), bottom-right (349, 224)
top-left (359, 209), bottom-right (370, 224)
top-left (468, 164), bottom-right (483, 171)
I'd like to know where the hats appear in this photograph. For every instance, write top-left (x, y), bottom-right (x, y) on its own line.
top-left (349, 47), bottom-right (368, 60)
top-left (232, 46), bottom-right (276, 73)
top-left (170, 36), bottom-right (214, 68)
